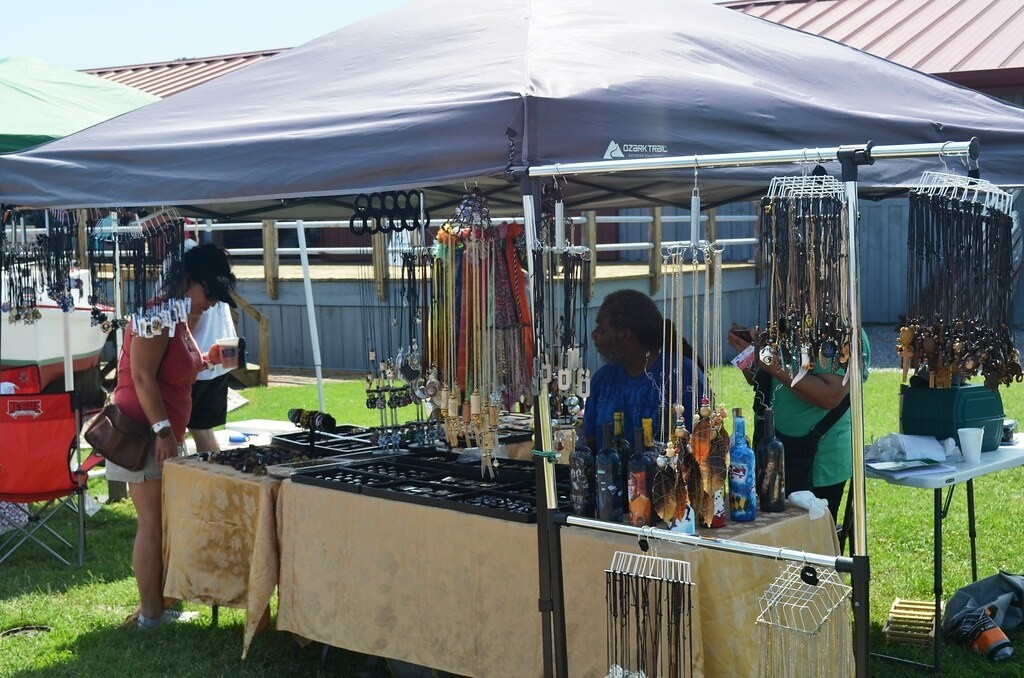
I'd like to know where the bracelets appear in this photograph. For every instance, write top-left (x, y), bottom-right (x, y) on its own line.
top-left (152, 419), bottom-right (174, 439)
top-left (207, 352), bottom-right (214, 371)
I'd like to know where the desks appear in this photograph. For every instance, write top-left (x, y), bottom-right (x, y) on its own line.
top-left (159, 429), bottom-right (858, 678)
top-left (849, 432), bottom-right (1024, 678)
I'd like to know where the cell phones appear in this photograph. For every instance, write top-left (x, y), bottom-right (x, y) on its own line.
top-left (732, 328), bottom-right (754, 343)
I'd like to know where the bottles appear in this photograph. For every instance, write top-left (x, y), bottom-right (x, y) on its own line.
top-left (755, 408), bottom-right (786, 511)
top-left (699, 485), bottom-right (725, 527)
top-left (595, 421), bottom-right (622, 522)
top-left (729, 416), bottom-right (756, 522)
top-left (614, 412), bottom-right (631, 514)
top-left (730, 408), bottom-right (751, 446)
top-left (628, 428), bottom-right (653, 525)
top-left (569, 417), bottom-right (594, 515)
top-left (641, 419), bottom-right (658, 452)
top-left (667, 503), bottom-right (695, 534)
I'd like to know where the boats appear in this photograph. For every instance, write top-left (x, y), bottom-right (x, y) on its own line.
top-left (0, 259), bottom-right (117, 396)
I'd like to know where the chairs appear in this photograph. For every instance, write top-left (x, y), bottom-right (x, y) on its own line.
top-left (0, 390), bottom-right (106, 569)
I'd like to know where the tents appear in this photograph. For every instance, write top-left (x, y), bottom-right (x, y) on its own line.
top-left (0, 0), bottom-right (1023, 678)
top-left (0, 55), bottom-right (326, 415)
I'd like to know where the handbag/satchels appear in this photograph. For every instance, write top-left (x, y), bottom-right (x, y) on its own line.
top-left (752, 419), bottom-right (820, 495)
top-left (85, 406), bottom-right (156, 470)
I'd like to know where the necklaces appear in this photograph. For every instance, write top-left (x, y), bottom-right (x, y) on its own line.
top-left (354, 219), bottom-right (591, 478)
top-left (604, 570), bottom-right (693, 678)
top-left (898, 190), bottom-right (1024, 387)
top-left (182, 324), bottom-right (192, 351)
top-left (654, 251), bottom-right (730, 528)
top-left (753, 197), bottom-right (849, 388)
top-left (3, 218), bottom-right (186, 334)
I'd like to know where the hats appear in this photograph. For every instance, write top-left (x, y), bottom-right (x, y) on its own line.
top-left (187, 264), bottom-right (238, 308)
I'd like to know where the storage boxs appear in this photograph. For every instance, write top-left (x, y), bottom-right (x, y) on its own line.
top-left (899, 384), bottom-right (1006, 453)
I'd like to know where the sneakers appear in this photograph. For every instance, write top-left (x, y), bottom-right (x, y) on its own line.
top-left (141, 610), bottom-right (196, 626)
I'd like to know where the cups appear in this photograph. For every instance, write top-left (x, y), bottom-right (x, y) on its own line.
top-left (958, 428), bottom-right (984, 462)
top-left (216, 337), bottom-right (239, 369)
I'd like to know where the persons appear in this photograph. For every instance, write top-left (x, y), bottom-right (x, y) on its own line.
top-left (115, 242), bottom-right (238, 626)
top-left (727, 321), bottom-right (871, 524)
top-left (140, 206), bottom-right (238, 453)
top-left (577, 290), bottom-right (704, 452)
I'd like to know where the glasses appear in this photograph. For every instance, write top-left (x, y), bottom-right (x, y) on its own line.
top-left (197, 277), bottom-right (219, 303)
